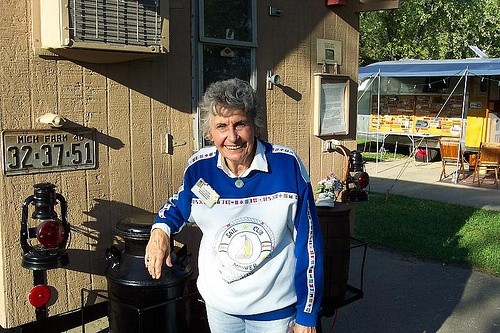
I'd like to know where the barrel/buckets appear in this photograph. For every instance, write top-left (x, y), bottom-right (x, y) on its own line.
top-left (316, 200), bottom-right (352, 305)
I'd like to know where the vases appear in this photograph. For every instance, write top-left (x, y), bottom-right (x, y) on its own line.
top-left (315, 195), bottom-right (335, 207)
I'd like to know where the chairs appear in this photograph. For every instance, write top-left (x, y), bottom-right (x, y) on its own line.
top-left (437, 138), bottom-right (467, 183)
top-left (469, 140), bottom-right (500, 186)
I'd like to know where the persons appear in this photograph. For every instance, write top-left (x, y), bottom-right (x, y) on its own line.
top-left (142, 80), bottom-right (325, 333)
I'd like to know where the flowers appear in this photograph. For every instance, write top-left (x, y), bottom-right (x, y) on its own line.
top-left (315, 171), bottom-right (343, 199)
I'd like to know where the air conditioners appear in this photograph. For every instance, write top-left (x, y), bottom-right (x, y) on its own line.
top-left (40, 0), bottom-right (171, 65)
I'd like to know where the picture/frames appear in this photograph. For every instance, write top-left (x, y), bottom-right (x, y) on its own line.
top-left (313, 70), bottom-right (351, 136)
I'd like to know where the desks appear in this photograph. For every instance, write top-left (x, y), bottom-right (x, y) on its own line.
top-left (367, 131), bottom-right (442, 167)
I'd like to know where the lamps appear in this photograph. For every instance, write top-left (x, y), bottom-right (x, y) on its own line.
top-left (342, 149), bottom-right (370, 204)
top-left (17, 181), bottom-right (72, 273)
